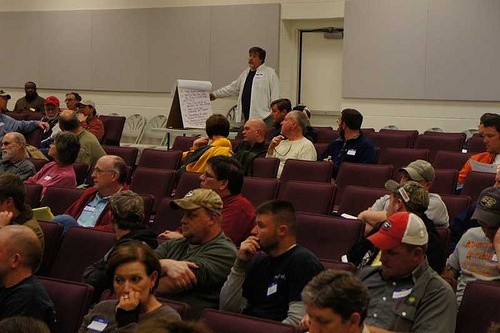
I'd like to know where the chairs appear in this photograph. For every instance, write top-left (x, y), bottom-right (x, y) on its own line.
top-left (0, 104), bottom-right (500, 333)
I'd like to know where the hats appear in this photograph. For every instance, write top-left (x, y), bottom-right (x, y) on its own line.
top-left (471, 185), bottom-right (500, 223)
top-left (368, 211), bottom-right (427, 251)
top-left (0, 90), bottom-right (11, 100)
top-left (400, 160), bottom-right (436, 183)
top-left (293, 106), bottom-right (311, 127)
top-left (385, 180), bottom-right (429, 213)
top-left (169, 188), bottom-right (223, 217)
top-left (44, 96), bottom-right (60, 106)
top-left (75, 97), bottom-right (95, 108)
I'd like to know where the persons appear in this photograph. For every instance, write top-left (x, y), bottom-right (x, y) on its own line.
top-left (149, 188), bottom-right (239, 322)
top-left (450, 113), bottom-right (500, 252)
top-left (77, 243), bottom-right (182, 333)
top-left (218, 199), bottom-right (325, 328)
top-left (81, 191), bottom-right (156, 315)
top-left (175, 114), bottom-right (233, 191)
top-left (0, 175), bottom-right (45, 272)
top-left (299, 211), bottom-right (458, 333)
top-left (0, 82), bottom-right (109, 200)
top-left (442, 187), bottom-right (500, 317)
top-left (233, 99), bottom-right (317, 181)
top-left (0, 224), bottom-right (57, 333)
top-left (301, 269), bottom-right (370, 333)
top-left (156, 154), bottom-right (258, 248)
top-left (210, 46), bottom-right (280, 127)
top-left (48, 154), bottom-right (132, 248)
top-left (343, 160), bottom-right (449, 274)
top-left (317, 108), bottom-right (377, 185)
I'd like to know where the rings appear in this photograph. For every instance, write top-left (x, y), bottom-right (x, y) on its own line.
top-left (124, 295), bottom-right (129, 299)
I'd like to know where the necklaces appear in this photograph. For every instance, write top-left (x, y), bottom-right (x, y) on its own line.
top-left (274, 138), bottom-right (293, 156)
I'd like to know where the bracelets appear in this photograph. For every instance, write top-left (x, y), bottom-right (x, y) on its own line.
top-left (233, 256), bottom-right (251, 273)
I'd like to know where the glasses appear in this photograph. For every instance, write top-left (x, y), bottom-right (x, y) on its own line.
top-left (91, 166), bottom-right (115, 174)
top-left (204, 171), bottom-right (217, 179)
top-left (0, 142), bottom-right (11, 146)
